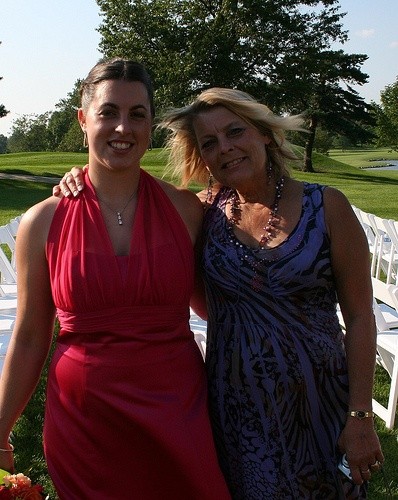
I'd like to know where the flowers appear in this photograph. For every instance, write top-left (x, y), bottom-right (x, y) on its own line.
top-left (0, 468), bottom-right (50, 500)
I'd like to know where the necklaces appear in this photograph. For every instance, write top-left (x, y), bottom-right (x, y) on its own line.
top-left (225, 174), bottom-right (289, 292)
top-left (95, 186), bottom-right (139, 226)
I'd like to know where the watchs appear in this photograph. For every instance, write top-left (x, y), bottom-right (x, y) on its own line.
top-left (347, 411), bottom-right (375, 420)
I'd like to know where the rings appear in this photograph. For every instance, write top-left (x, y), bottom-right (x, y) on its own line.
top-left (370, 461), bottom-right (380, 467)
top-left (361, 469), bottom-right (370, 474)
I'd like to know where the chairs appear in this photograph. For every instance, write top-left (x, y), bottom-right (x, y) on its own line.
top-left (335, 204), bottom-right (398, 429)
top-left (0, 214), bottom-right (21, 373)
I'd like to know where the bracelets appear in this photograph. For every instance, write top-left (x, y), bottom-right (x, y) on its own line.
top-left (0, 443), bottom-right (15, 452)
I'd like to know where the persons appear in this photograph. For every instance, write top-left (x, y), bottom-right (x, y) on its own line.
top-left (0, 58), bottom-right (233, 500)
top-left (52, 87), bottom-right (386, 500)
